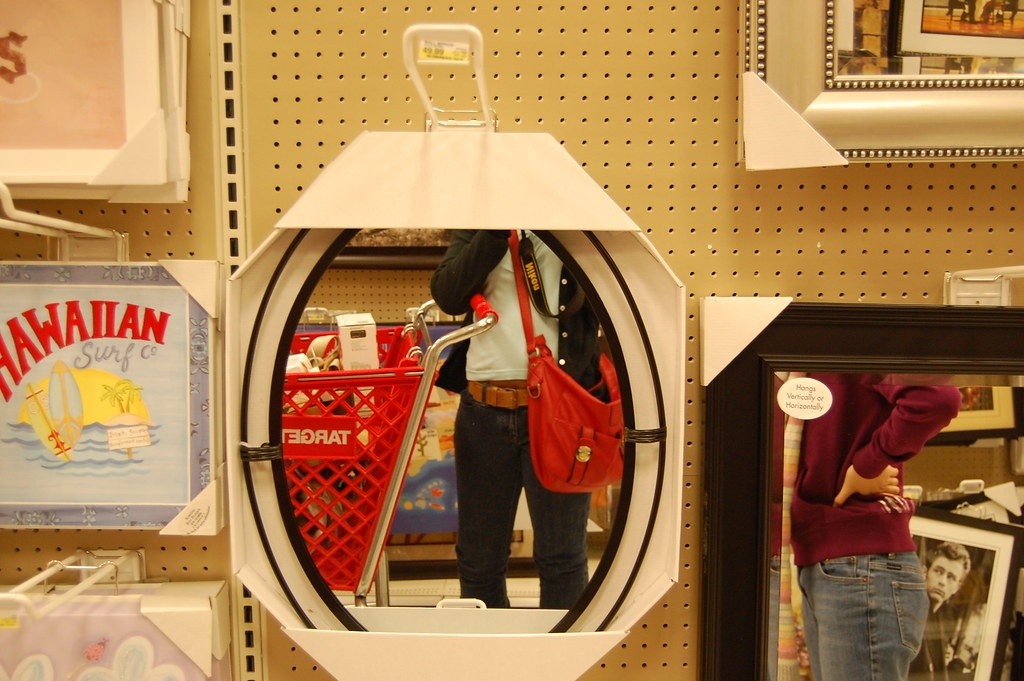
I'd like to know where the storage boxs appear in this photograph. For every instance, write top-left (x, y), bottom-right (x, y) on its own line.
top-left (0, 581), bottom-right (230, 681)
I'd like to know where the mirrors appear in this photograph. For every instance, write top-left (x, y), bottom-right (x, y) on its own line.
top-left (700, 297), bottom-right (1024, 681)
top-left (239, 227), bottom-right (667, 631)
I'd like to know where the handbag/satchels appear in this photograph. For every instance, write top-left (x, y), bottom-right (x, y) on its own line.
top-left (526, 336), bottom-right (624, 495)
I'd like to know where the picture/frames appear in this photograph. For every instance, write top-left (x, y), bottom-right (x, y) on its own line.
top-left (924, 386), bottom-right (1024, 447)
top-left (737, 0), bottom-right (1024, 172)
top-left (0, 0), bottom-right (229, 537)
top-left (908, 480), bottom-right (1024, 681)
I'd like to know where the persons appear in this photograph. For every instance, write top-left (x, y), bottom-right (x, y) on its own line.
top-left (945, 0), bottom-right (1020, 23)
top-left (429, 229), bottom-right (625, 608)
top-left (909, 540), bottom-right (971, 681)
top-left (776, 374), bottom-right (961, 681)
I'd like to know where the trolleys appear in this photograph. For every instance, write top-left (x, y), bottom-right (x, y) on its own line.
top-left (279, 293), bottom-right (498, 608)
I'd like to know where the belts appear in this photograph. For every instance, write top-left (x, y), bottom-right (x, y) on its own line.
top-left (468, 380), bottom-right (534, 410)
